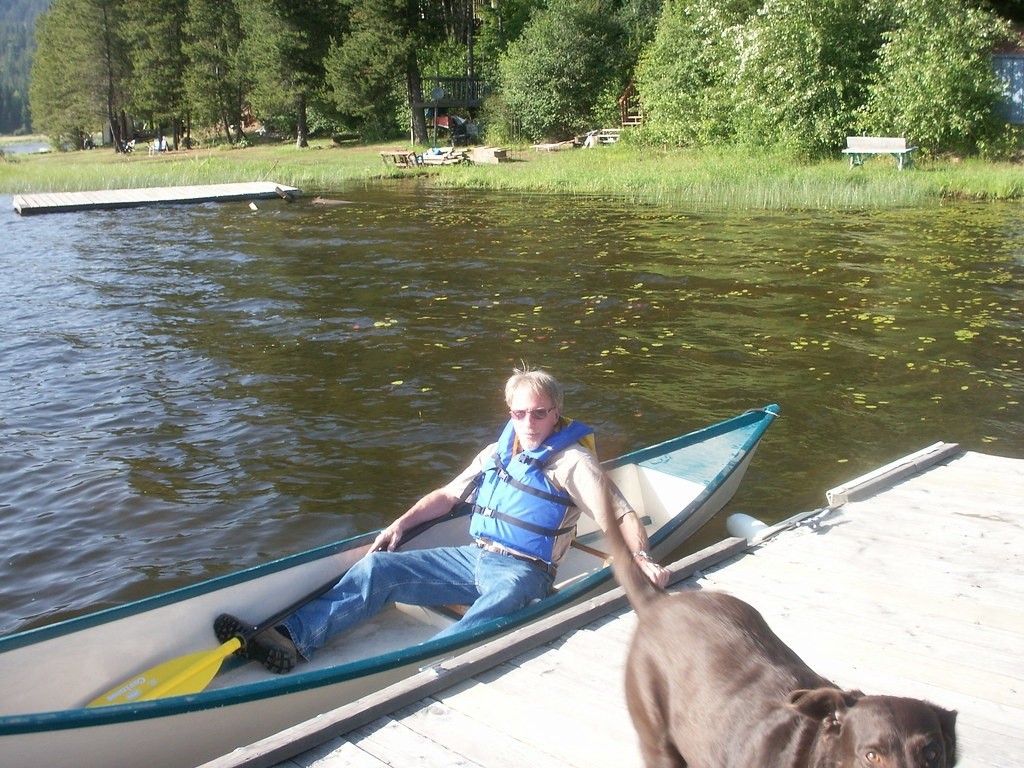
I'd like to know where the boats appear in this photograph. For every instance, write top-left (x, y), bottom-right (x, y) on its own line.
top-left (1, 399), bottom-right (782, 768)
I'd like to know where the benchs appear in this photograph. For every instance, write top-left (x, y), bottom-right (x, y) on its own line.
top-left (381, 151), bottom-right (425, 169)
top-left (148, 138), bottom-right (168, 155)
top-left (841, 131), bottom-right (919, 170)
top-left (445, 587), bottom-right (560, 618)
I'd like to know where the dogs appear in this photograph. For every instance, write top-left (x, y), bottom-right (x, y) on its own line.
top-left (591, 472), bottom-right (960, 768)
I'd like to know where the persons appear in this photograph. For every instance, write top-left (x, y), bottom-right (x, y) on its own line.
top-left (213, 371), bottom-right (669, 674)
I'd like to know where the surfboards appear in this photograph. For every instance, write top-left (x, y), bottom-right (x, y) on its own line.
top-left (84, 499), bottom-right (469, 707)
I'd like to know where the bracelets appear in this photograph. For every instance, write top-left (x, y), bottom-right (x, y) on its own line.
top-left (632, 551), bottom-right (653, 561)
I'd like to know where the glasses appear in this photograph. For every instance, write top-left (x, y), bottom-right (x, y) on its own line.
top-left (508, 405), bottom-right (556, 420)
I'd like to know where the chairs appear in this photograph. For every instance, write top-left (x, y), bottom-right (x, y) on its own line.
top-left (130, 140), bottom-right (136, 152)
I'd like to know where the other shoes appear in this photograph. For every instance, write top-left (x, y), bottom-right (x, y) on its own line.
top-left (213, 613), bottom-right (297, 674)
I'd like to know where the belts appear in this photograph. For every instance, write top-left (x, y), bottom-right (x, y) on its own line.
top-left (475, 541), bottom-right (557, 578)
